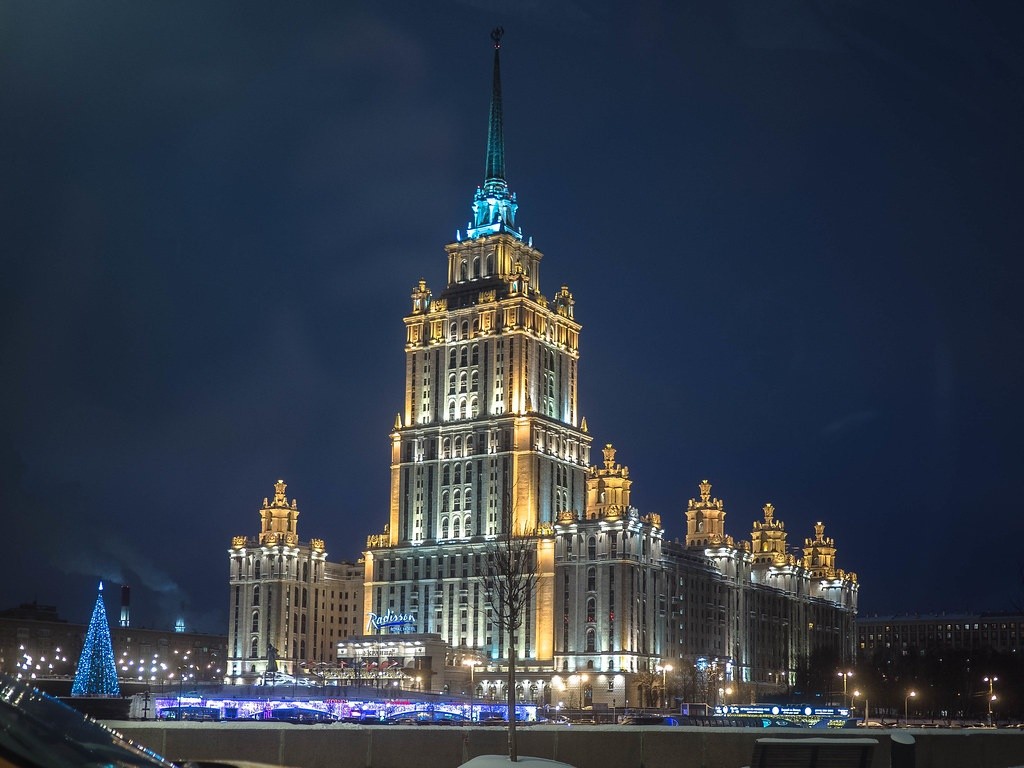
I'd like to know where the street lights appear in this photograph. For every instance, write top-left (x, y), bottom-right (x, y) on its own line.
top-left (838, 670), bottom-right (852, 708)
top-left (984, 676), bottom-right (998, 727)
top-left (905, 692), bottom-right (916, 726)
